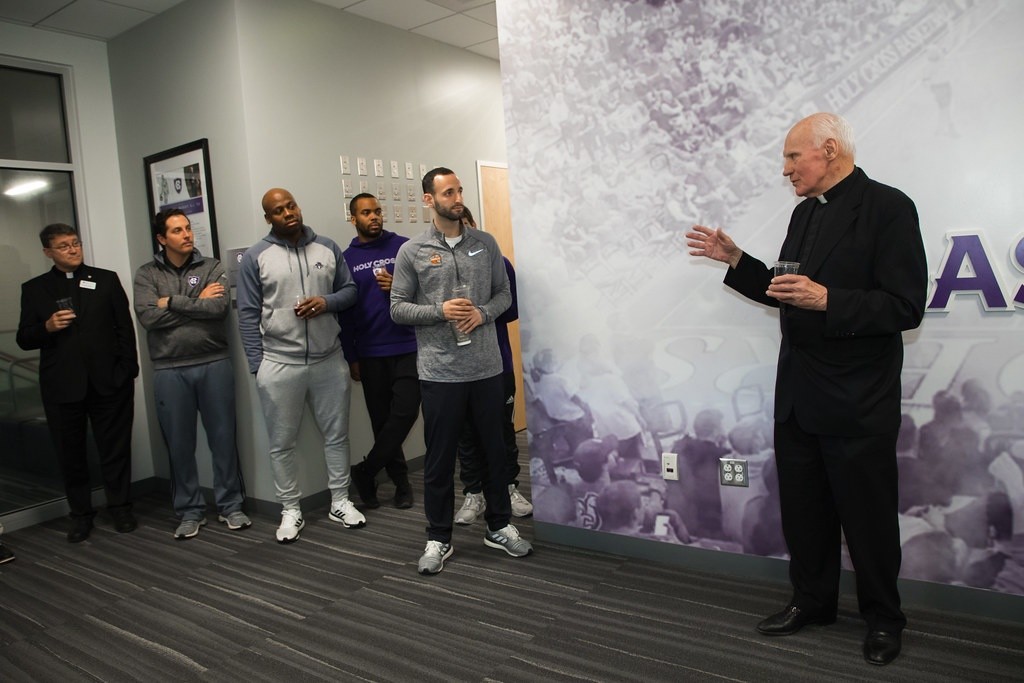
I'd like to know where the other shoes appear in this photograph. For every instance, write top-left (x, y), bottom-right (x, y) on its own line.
top-left (394, 483), bottom-right (414, 509)
top-left (114, 509), bottom-right (138, 533)
top-left (350, 456), bottom-right (380, 508)
top-left (0, 544), bottom-right (16, 564)
top-left (67, 518), bottom-right (95, 543)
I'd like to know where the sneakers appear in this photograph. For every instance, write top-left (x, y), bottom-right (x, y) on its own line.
top-left (417, 540), bottom-right (453, 575)
top-left (484, 525), bottom-right (534, 557)
top-left (454, 491), bottom-right (487, 524)
top-left (173, 516), bottom-right (208, 539)
top-left (508, 484), bottom-right (533, 517)
top-left (329, 498), bottom-right (367, 529)
top-left (218, 511), bottom-right (252, 530)
top-left (276, 508), bottom-right (306, 544)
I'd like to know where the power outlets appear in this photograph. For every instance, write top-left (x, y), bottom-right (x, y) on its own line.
top-left (660, 452), bottom-right (680, 482)
top-left (719, 457), bottom-right (749, 488)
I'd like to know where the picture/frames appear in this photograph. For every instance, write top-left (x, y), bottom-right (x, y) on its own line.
top-left (143, 138), bottom-right (220, 261)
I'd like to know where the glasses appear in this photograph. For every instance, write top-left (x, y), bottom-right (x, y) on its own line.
top-left (49, 239), bottom-right (82, 251)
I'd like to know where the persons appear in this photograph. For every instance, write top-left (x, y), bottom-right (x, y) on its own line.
top-left (495, 0), bottom-right (1024, 597)
top-left (337, 192), bottom-right (422, 509)
top-left (236, 188), bottom-right (368, 545)
top-left (133, 209), bottom-right (252, 540)
top-left (15, 223), bottom-right (140, 544)
top-left (684, 112), bottom-right (929, 667)
top-left (390, 167), bottom-right (535, 575)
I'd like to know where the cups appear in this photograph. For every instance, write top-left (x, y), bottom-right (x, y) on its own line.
top-left (57, 298), bottom-right (74, 319)
top-left (371, 263), bottom-right (386, 280)
top-left (452, 285), bottom-right (471, 306)
top-left (449, 320), bottom-right (471, 346)
top-left (774, 261), bottom-right (800, 299)
top-left (292, 295), bottom-right (307, 318)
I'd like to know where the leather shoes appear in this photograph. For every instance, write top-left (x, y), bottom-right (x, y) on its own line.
top-left (756, 599), bottom-right (839, 636)
top-left (862, 627), bottom-right (903, 665)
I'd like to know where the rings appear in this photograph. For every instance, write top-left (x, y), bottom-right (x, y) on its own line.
top-left (310, 307), bottom-right (316, 312)
